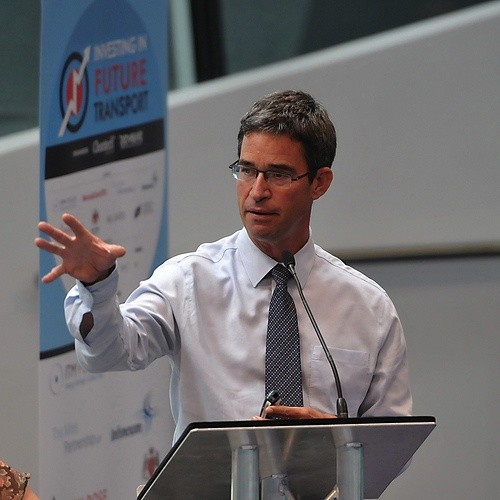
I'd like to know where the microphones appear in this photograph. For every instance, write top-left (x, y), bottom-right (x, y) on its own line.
top-left (282, 250), bottom-right (348, 418)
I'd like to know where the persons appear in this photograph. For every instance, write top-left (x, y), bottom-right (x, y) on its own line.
top-left (33, 89), bottom-right (414, 451)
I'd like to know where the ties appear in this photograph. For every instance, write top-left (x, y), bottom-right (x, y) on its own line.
top-left (264, 264), bottom-right (303, 421)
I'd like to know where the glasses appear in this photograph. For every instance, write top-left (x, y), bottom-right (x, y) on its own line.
top-left (228, 159), bottom-right (319, 190)
